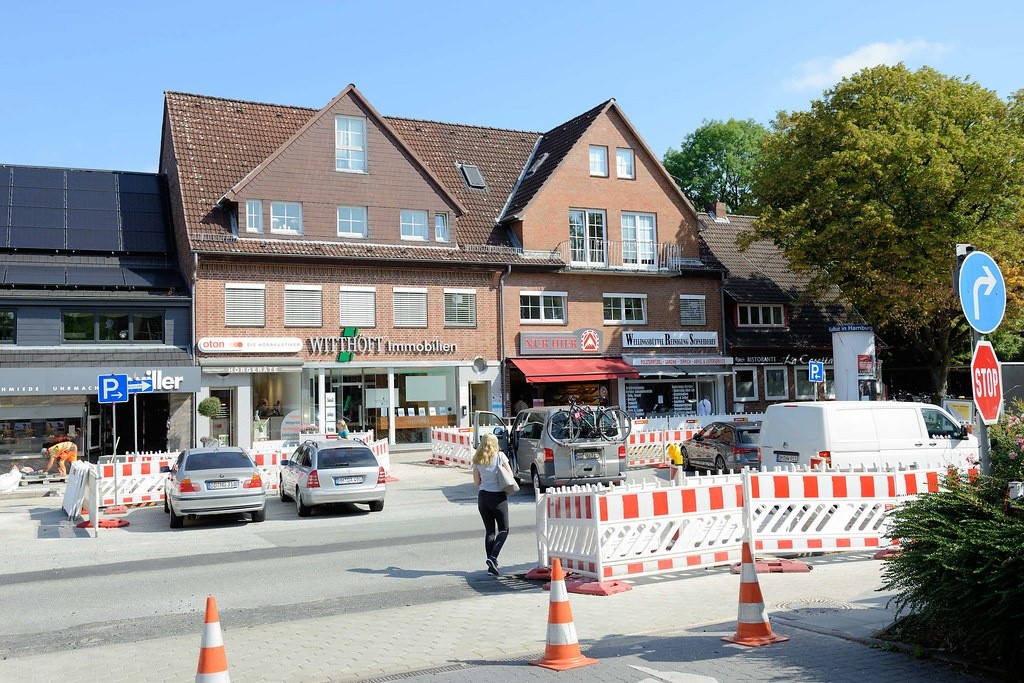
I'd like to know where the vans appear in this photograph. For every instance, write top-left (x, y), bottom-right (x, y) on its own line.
top-left (757, 401), bottom-right (980, 470)
top-left (473, 404), bottom-right (628, 493)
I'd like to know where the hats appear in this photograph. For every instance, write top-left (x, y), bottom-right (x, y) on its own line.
top-left (41, 448), bottom-right (47, 459)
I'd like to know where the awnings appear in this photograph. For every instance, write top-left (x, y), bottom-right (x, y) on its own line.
top-left (507, 356), bottom-right (640, 383)
top-left (630, 366), bottom-right (737, 377)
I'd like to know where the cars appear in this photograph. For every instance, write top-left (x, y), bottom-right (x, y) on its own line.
top-left (160, 446), bottom-right (267, 528)
top-left (679, 422), bottom-right (760, 473)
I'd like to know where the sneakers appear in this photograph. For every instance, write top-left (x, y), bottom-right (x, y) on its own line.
top-left (486, 556), bottom-right (500, 576)
top-left (487, 567), bottom-right (494, 576)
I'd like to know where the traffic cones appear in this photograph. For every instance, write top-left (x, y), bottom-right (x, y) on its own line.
top-left (528, 557), bottom-right (600, 671)
top-left (194, 594), bottom-right (231, 683)
top-left (719, 542), bottom-right (790, 646)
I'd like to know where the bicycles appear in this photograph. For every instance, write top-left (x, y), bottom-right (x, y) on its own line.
top-left (546, 395), bottom-right (632, 446)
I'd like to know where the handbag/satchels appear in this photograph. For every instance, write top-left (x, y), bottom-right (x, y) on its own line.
top-left (497, 452), bottom-right (520, 495)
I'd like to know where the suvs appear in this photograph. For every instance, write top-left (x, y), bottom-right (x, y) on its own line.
top-left (279, 438), bottom-right (386, 517)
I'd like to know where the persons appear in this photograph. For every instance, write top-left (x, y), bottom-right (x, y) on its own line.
top-left (472, 433), bottom-right (514, 576)
top-left (698, 396), bottom-right (711, 416)
top-left (336, 420), bottom-right (349, 440)
top-left (42, 442), bottom-right (77, 477)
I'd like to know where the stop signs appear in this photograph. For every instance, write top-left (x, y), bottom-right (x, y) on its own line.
top-left (971, 341), bottom-right (1003, 424)
